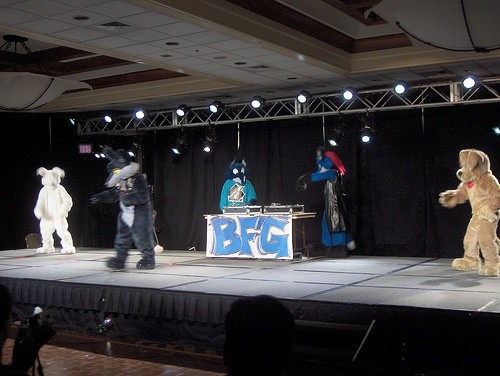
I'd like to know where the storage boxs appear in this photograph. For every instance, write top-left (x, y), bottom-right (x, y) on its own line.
top-left (264, 204), bottom-right (304, 215)
top-left (222, 207), bottom-right (262, 214)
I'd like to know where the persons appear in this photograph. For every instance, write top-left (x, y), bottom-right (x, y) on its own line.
top-left (220, 293), bottom-right (307, 376)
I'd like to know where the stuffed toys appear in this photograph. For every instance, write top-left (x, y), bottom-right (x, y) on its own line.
top-left (219, 151), bottom-right (258, 214)
top-left (89, 146), bottom-right (156, 270)
top-left (32, 167), bottom-right (77, 254)
top-left (438, 148), bottom-right (500, 277)
top-left (296, 146), bottom-right (357, 258)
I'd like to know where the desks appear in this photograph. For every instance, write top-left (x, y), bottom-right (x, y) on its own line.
top-left (204, 214), bottom-right (310, 264)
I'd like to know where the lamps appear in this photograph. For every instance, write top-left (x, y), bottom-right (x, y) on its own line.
top-left (463, 71), bottom-right (478, 89)
top-left (128, 129), bottom-right (140, 156)
top-left (394, 80), bottom-right (408, 94)
top-left (104, 113), bottom-right (115, 122)
top-left (203, 123), bottom-right (215, 152)
top-left (171, 126), bottom-right (186, 154)
top-left (177, 104), bottom-right (188, 116)
top-left (94, 140), bottom-right (113, 158)
top-left (251, 95), bottom-right (264, 108)
top-left (343, 86), bottom-right (356, 100)
top-left (0, 32), bottom-right (94, 111)
top-left (328, 113), bottom-right (344, 147)
top-left (209, 101), bottom-right (224, 113)
top-left (298, 90), bottom-right (311, 103)
top-left (135, 108), bottom-right (148, 119)
top-left (362, 111), bottom-right (372, 143)
top-left (70, 118), bottom-right (79, 124)
